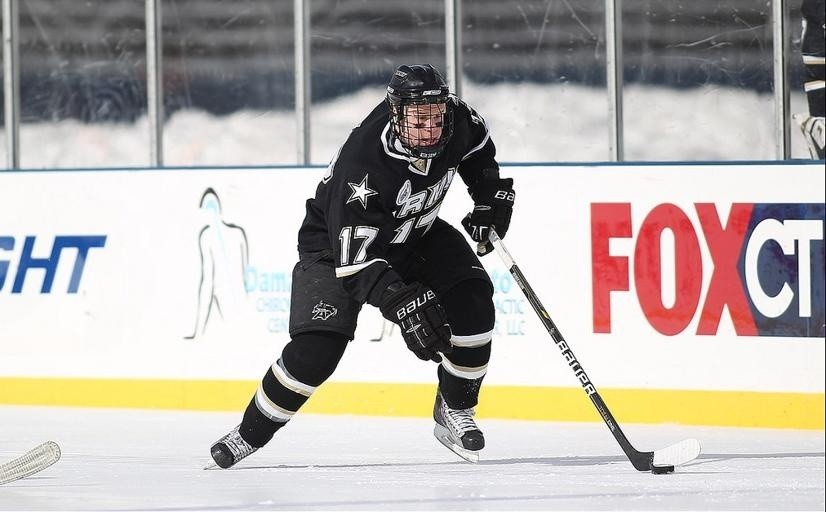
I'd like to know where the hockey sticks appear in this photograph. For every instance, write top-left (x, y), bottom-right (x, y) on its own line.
top-left (1, 442), bottom-right (60, 485)
top-left (488, 227), bottom-right (700, 471)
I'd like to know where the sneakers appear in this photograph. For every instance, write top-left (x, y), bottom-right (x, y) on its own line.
top-left (210, 425), bottom-right (259, 468)
top-left (434, 389), bottom-right (485, 450)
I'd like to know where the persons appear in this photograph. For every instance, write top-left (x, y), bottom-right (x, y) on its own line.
top-left (797, 0), bottom-right (825, 164)
top-left (207, 64), bottom-right (517, 470)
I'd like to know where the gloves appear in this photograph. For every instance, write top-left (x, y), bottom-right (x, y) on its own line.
top-left (381, 282), bottom-right (452, 362)
top-left (468, 178), bottom-right (515, 256)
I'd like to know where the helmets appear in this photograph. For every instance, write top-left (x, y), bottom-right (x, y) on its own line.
top-left (387, 64), bottom-right (454, 159)
